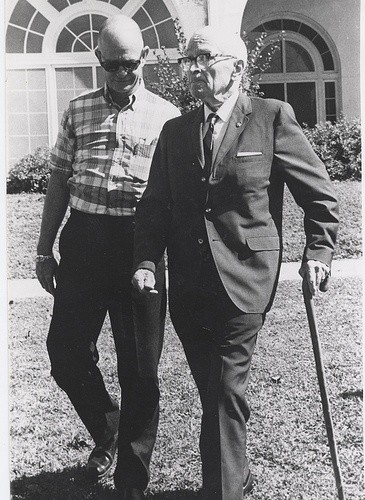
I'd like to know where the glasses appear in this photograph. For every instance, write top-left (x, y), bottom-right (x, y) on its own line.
top-left (99, 58), bottom-right (141, 73)
top-left (177, 52), bottom-right (234, 71)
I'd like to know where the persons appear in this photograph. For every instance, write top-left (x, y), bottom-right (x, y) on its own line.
top-left (130, 26), bottom-right (339, 500)
top-left (37, 14), bottom-right (182, 500)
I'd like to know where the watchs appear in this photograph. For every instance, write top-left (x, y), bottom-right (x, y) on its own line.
top-left (35, 255), bottom-right (53, 262)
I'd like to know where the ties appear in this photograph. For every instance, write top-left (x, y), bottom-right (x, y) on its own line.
top-left (203, 116), bottom-right (218, 178)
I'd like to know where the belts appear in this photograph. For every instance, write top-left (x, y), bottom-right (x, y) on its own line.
top-left (71, 209), bottom-right (132, 227)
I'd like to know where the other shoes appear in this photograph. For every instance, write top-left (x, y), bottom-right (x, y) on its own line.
top-left (242, 471), bottom-right (255, 495)
top-left (86, 433), bottom-right (118, 477)
top-left (115, 485), bottom-right (146, 500)
